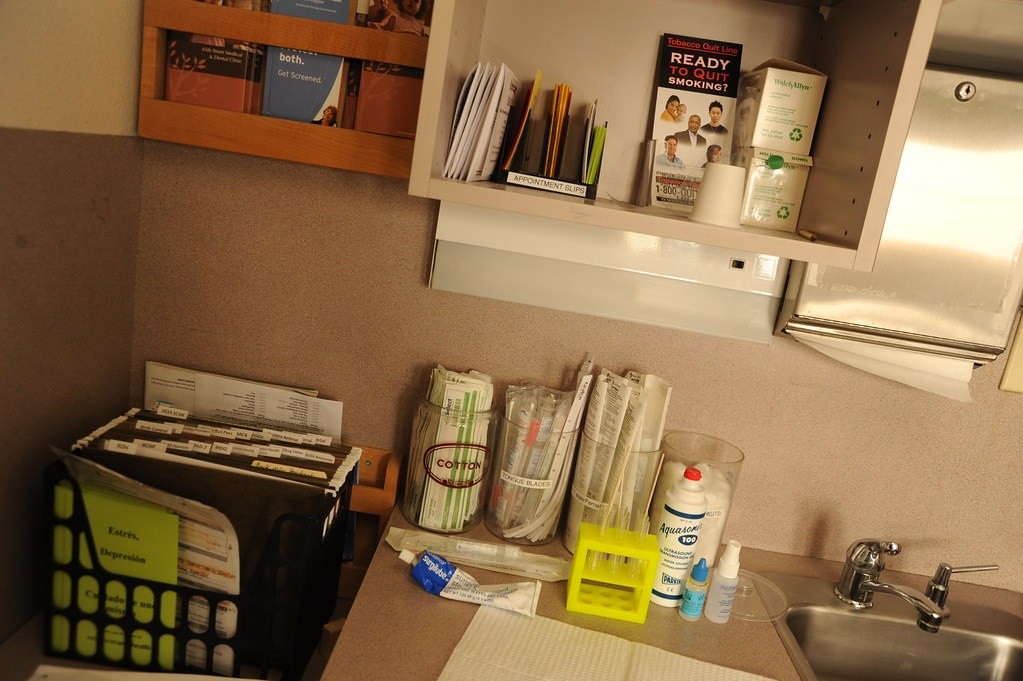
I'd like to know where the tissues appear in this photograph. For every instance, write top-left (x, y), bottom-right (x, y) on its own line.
top-left (772, 59), bottom-right (1023, 404)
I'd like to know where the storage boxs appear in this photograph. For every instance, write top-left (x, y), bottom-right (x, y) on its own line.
top-left (732, 55), bottom-right (829, 157)
top-left (731, 148), bottom-right (813, 233)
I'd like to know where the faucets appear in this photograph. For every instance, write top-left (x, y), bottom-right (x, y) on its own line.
top-left (832, 537), bottom-right (944, 634)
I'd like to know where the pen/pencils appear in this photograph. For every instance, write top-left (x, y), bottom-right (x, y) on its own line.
top-left (798, 229), bottom-right (819, 241)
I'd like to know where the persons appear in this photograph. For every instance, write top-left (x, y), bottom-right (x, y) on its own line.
top-left (384, 0), bottom-right (425, 36)
top-left (368, 0), bottom-right (391, 30)
top-left (674, 115), bottom-right (706, 146)
top-left (662, 95), bottom-right (687, 123)
top-left (700, 100), bottom-right (728, 134)
top-left (311, 106), bottom-right (337, 126)
top-left (701, 145), bottom-right (722, 168)
top-left (657, 135), bottom-right (683, 168)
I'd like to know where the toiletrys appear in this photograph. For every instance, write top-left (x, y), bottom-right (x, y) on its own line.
top-left (398, 547), bottom-right (542, 618)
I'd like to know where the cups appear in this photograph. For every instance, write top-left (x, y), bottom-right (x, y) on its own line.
top-left (650, 431), bottom-right (745, 569)
top-left (485, 409), bottom-right (582, 547)
top-left (688, 161), bottom-right (747, 228)
top-left (562, 427), bottom-right (665, 556)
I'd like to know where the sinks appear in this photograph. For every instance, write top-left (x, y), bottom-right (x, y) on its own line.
top-left (752, 569), bottom-right (1023, 681)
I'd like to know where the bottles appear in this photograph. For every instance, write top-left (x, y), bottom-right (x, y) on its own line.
top-left (704, 541), bottom-right (741, 624)
top-left (655, 467), bottom-right (708, 608)
top-left (679, 558), bottom-right (708, 621)
top-left (402, 391), bottom-right (501, 535)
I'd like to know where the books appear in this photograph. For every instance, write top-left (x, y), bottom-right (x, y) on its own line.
top-left (164, 0), bottom-right (436, 138)
